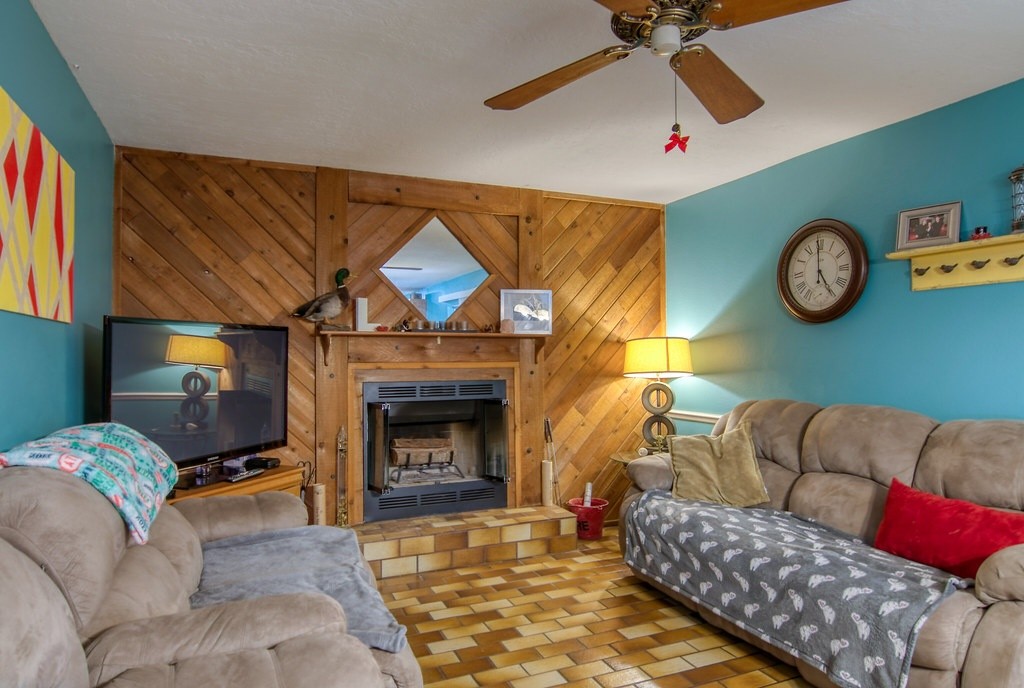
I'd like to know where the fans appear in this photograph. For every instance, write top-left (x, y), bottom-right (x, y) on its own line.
top-left (484, 0), bottom-right (852, 126)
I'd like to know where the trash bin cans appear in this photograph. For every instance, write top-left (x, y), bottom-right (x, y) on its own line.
top-left (566, 497), bottom-right (609, 540)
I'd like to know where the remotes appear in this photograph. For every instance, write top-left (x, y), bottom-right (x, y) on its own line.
top-left (226, 467), bottom-right (265, 483)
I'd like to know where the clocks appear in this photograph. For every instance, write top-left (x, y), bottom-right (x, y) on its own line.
top-left (777, 218), bottom-right (869, 323)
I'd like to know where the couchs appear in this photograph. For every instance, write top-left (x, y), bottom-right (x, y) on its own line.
top-left (0, 440), bottom-right (427, 688)
top-left (621, 397), bottom-right (1024, 688)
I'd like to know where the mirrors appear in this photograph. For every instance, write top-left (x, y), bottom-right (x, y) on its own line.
top-left (373, 209), bottom-right (500, 331)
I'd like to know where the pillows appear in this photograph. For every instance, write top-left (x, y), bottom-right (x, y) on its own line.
top-left (665, 420), bottom-right (770, 508)
top-left (874, 478), bottom-right (1024, 579)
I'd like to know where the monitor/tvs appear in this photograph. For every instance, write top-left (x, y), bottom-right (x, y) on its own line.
top-left (98, 314), bottom-right (289, 490)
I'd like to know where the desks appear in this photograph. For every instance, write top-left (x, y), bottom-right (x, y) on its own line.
top-left (164, 465), bottom-right (307, 507)
top-left (610, 449), bottom-right (677, 468)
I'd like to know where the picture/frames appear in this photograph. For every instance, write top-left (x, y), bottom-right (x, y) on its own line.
top-left (893, 201), bottom-right (962, 252)
top-left (500, 288), bottom-right (553, 336)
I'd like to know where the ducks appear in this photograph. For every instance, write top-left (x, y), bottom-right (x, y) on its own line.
top-left (288, 267), bottom-right (359, 325)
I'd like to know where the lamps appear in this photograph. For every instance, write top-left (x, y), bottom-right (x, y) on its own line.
top-left (650, 25), bottom-right (690, 153)
top-left (623, 336), bottom-right (696, 455)
